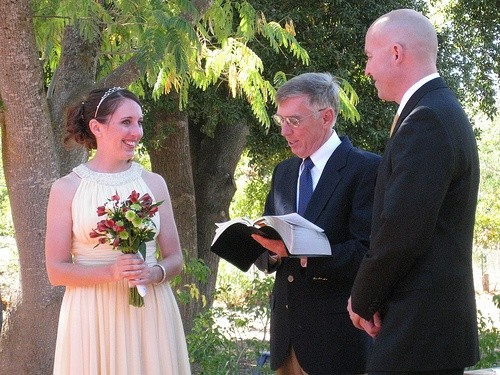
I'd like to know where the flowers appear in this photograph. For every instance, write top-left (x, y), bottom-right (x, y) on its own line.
top-left (89, 189), bottom-right (165, 308)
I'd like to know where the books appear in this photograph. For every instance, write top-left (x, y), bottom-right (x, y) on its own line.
top-left (208, 211), bottom-right (333, 273)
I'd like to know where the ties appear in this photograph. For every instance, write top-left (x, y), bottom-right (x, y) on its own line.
top-left (297, 156), bottom-right (315, 217)
top-left (390, 113), bottom-right (400, 137)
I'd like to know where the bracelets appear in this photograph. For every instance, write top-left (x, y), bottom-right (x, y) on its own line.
top-left (149, 263), bottom-right (166, 285)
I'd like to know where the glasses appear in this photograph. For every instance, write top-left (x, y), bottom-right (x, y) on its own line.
top-left (273, 109), bottom-right (322, 128)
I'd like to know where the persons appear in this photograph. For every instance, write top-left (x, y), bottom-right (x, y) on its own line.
top-left (42, 86), bottom-right (192, 375)
top-left (254, 71), bottom-right (382, 374)
top-left (344, 9), bottom-right (480, 374)
